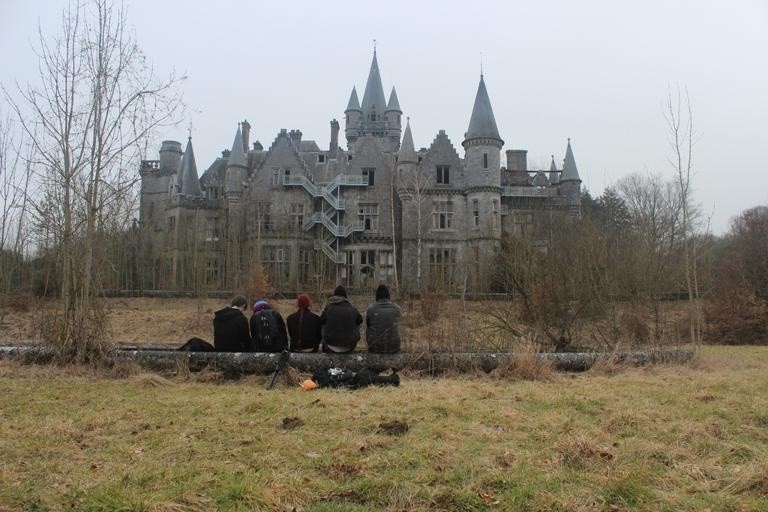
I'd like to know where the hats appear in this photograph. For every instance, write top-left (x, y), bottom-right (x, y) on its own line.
top-left (334, 285), bottom-right (346, 297)
top-left (375, 284), bottom-right (391, 301)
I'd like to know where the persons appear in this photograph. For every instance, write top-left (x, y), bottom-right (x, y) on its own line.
top-left (212, 296), bottom-right (249, 352)
top-left (365, 285), bottom-right (402, 354)
top-left (320, 285), bottom-right (363, 354)
top-left (286, 293), bottom-right (320, 353)
top-left (250, 300), bottom-right (288, 352)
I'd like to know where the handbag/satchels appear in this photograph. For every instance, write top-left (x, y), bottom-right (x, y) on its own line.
top-left (313, 367), bottom-right (400, 390)
top-left (178, 339), bottom-right (214, 353)
